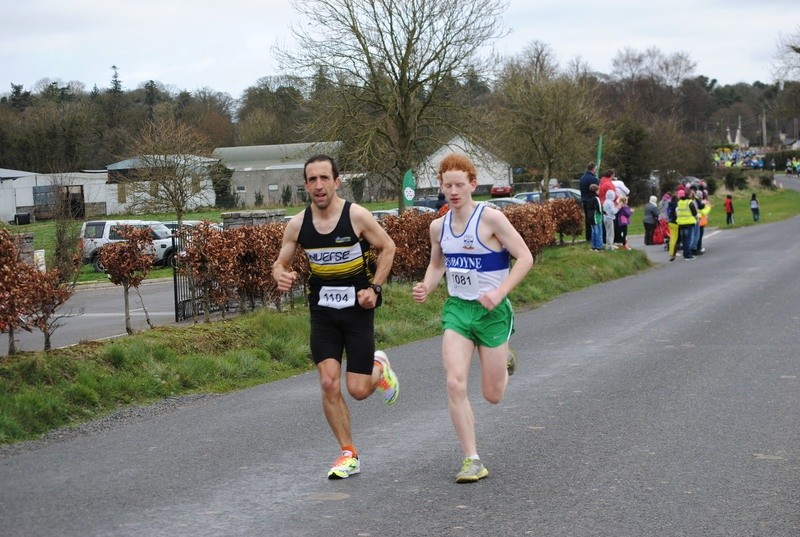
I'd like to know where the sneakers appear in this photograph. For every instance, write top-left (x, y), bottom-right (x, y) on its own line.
top-left (373, 350), bottom-right (398, 405)
top-left (454, 457), bottom-right (489, 482)
top-left (327, 449), bottom-right (360, 478)
top-left (507, 350), bottom-right (514, 376)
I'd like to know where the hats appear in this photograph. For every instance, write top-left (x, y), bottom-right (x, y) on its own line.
top-left (590, 184), bottom-right (599, 190)
top-left (678, 190), bottom-right (685, 197)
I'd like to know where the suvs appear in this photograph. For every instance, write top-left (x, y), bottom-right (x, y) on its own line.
top-left (76, 219), bottom-right (196, 275)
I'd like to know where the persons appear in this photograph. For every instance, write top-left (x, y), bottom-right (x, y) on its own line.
top-left (273, 154), bottom-right (401, 480)
top-left (580, 162), bottom-right (636, 253)
top-left (553, 184), bottom-right (559, 190)
top-left (435, 192), bottom-right (449, 213)
top-left (749, 193), bottom-right (760, 222)
top-left (708, 147), bottom-right (800, 181)
top-left (723, 193), bottom-right (735, 226)
top-left (643, 175), bottom-right (715, 262)
top-left (413, 152), bottom-right (533, 483)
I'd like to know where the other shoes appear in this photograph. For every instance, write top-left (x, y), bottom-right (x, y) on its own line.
top-left (593, 244), bottom-right (632, 251)
top-left (700, 248), bottom-right (705, 252)
top-left (685, 256), bottom-right (696, 260)
top-left (691, 250), bottom-right (704, 255)
top-left (669, 257), bottom-right (675, 261)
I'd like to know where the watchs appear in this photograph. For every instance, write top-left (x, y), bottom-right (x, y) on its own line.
top-left (368, 283), bottom-right (383, 295)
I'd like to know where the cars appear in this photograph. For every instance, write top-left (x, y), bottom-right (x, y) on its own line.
top-left (540, 178), bottom-right (559, 189)
top-left (490, 180), bottom-right (513, 198)
top-left (162, 219), bottom-right (224, 245)
top-left (643, 168), bottom-right (709, 198)
top-left (371, 195), bottom-right (450, 220)
top-left (477, 187), bottom-right (581, 212)
